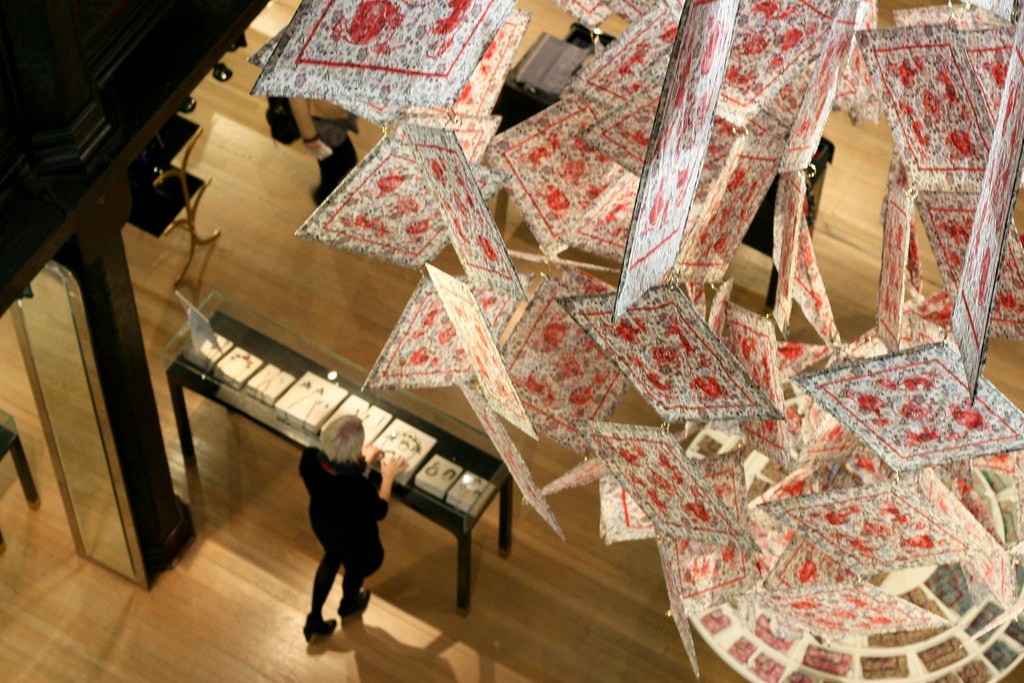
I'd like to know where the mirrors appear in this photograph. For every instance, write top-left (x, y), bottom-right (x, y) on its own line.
top-left (12, 261), bottom-right (150, 591)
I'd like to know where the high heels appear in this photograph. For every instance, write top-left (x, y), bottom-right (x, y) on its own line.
top-left (303, 612), bottom-right (337, 642)
top-left (338, 588), bottom-right (371, 626)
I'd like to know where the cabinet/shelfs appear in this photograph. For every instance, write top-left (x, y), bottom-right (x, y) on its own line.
top-left (124, 113), bottom-right (221, 290)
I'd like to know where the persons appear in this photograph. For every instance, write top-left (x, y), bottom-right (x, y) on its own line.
top-left (297, 415), bottom-right (407, 644)
top-left (289, 96), bottom-right (362, 210)
